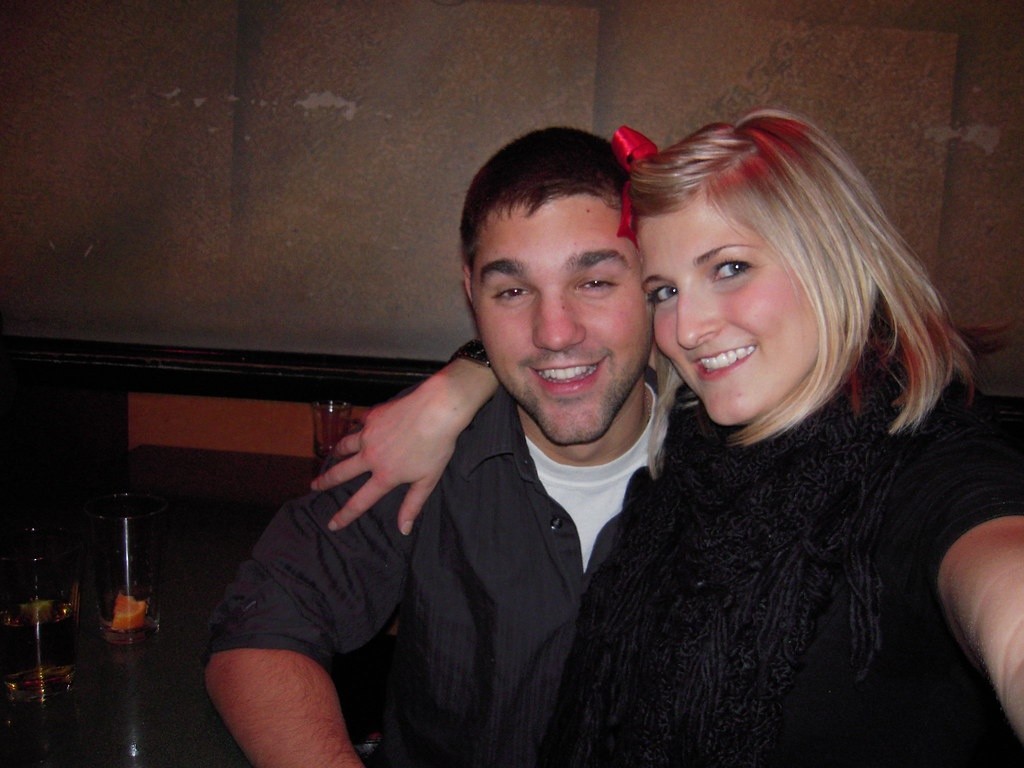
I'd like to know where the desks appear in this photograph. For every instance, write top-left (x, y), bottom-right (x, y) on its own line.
top-left (0, 483), bottom-right (395, 768)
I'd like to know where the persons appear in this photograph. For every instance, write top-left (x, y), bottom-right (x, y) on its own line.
top-left (201, 104), bottom-right (1024, 768)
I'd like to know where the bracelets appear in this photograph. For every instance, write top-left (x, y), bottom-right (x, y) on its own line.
top-left (448, 339), bottom-right (490, 367)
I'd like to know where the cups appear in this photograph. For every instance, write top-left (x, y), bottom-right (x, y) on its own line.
top-left (0, 522), bottom-right (86, 706)
top-left (81, 491), bottom-right (172, 645)
top-left (310, 401), bottom-right (352, 468)
